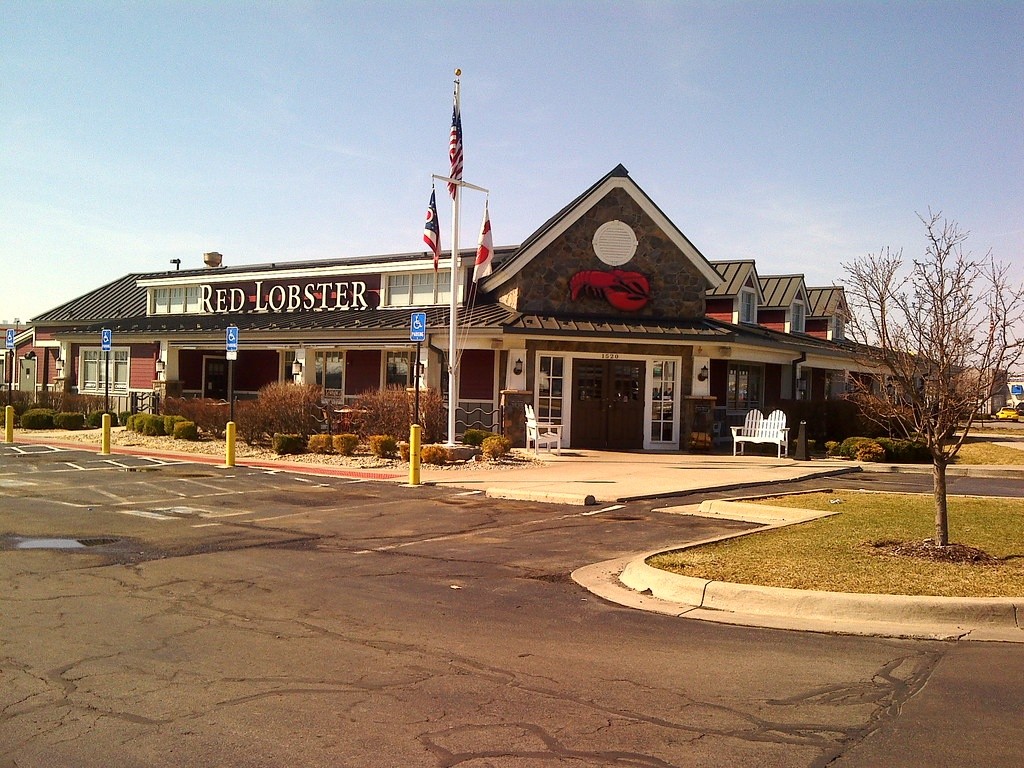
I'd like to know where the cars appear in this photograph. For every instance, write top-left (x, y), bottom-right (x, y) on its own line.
top-left (996, 407), bottom-right (1020, 423)
top-left (1015, 402), bottom-right (1024, 416)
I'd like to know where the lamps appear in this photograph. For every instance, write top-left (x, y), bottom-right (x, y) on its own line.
top-left (56, 356), bottom-right (65, 370)
top-left (156, 359), bottom-right (166, 373)
top-left (415, 361), bottom-right (425, 378)
top-left (514, 358), bottom-right (523, 375)
top-left (292, 358), bottom-right (303, 375)
top-left (698, 366), bottom-right (708, 381)
top-left (797, 376), bottom-right (808, 391)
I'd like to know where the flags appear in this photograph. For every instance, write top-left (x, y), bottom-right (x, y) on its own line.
top-left (446, 87), bottom-right (463, 200)
top-left (422, 186), bottom-right (442, 273)
top-left (472, 199), bottom-right (494, 283)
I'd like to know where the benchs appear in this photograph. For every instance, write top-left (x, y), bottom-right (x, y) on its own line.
top-left (730, 409), bottom-right (791, 459)
top-left (525, 403), bottom-right (565, 455)
